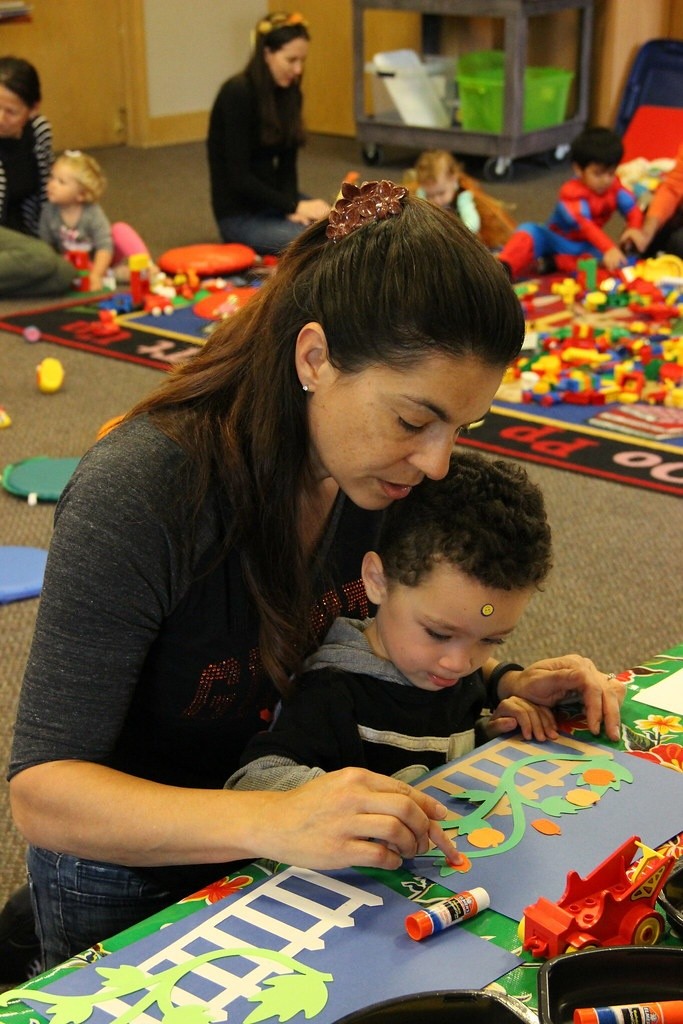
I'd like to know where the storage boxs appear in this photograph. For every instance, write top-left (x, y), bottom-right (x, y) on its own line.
top-left (452, 49), bottom-right (575, 133)
top-left (364, 51), bottom-right (461, 133)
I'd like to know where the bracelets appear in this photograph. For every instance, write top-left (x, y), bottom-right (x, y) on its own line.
top-left (487, 661), bottom-right (523, 709)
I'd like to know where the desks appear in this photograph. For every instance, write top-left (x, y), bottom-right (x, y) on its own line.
top-left (0, 649), bottom-right (678, 1024)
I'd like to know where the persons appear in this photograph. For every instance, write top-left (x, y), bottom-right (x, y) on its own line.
top-left (491, 130), bottom-right (647, 279)
top-left (220, 445), bottom-right (563, 863)
top-left (4, 177), bottom-right (628, 972)
top-left (0, 53), bottom-right (76, 293)
top-left (416, 152), bottom-right (518, 255)
top-left (39, 152), bottom-right (177, 302)
top-left (209, 10), bottom-right (344, 262)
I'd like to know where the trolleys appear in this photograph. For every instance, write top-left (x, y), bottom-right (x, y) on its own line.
top-left (351, 0), bottom-right (598, 183)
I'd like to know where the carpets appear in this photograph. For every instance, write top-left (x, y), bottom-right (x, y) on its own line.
top-left (0, 233), bottom-right (683, 500)
